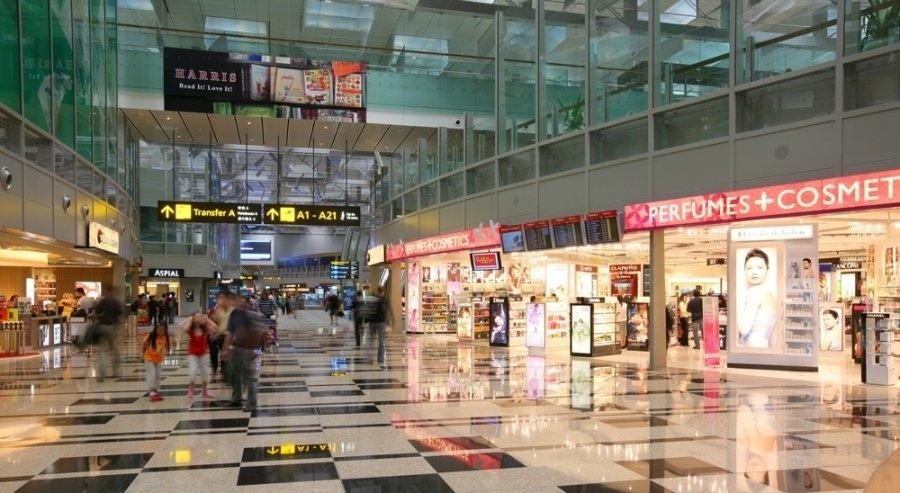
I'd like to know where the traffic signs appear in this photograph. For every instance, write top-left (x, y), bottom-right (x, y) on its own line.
top-left (157, 199), bottom-right (362, 227)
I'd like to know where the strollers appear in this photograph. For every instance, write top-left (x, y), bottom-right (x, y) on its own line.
top-left (261, 315), bottom-right (279, 353)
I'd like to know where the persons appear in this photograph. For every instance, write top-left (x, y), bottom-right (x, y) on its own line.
top-left (458, 306), bottom-right (472, 338)
top-left (627, 312), bottom-right (642, 339)
top-left (530, 294), bottom-right (556, 302)
top-left (666, 289), bottom-right (727, 350)
top-left (0, 295), bottom-right (19, 321)
top-left (410, 263), bottom-right (530, 295)
top-left (63, 285), bottom-right (392, 411)
top-left (821, 310), bottom-right (842, 350)
top-left (736, 249), bottom-right (777, 349)
top-left (409, 309), bottom-right (417, 330)
top-left (491, 303), bottom-right (507, 343)
top-left (803, 258), bottom-right (813, 279)
top-left (528, 304), bottom-right (541, 333)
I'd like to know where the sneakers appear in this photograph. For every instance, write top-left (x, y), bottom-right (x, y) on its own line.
top-left (149, 394), bottom-right (163, 402)
top-left (185, 393), bottom-right (195, 402)
top-left (201, 391), bottom-right (213, 401)
